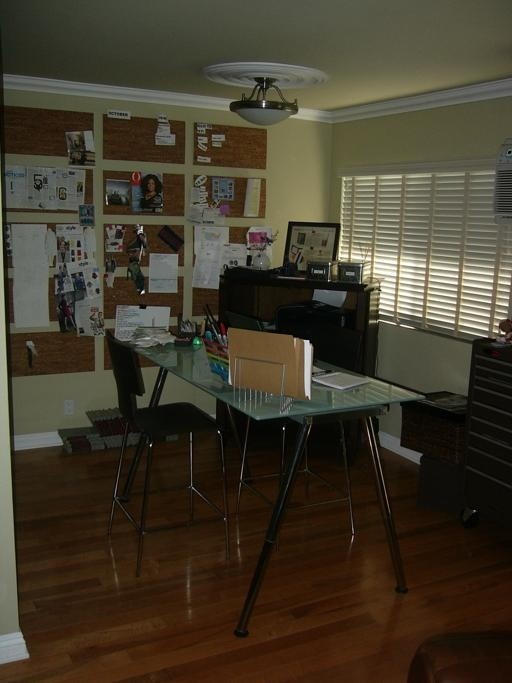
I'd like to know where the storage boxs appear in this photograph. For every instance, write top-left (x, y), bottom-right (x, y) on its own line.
top-left (400, 391), bottom-right (468, 456)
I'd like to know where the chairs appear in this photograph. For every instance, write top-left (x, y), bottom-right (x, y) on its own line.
top-left (105, 330), bottom-right (230, 577)
top-left (236, 327), bottom-right (364, 553)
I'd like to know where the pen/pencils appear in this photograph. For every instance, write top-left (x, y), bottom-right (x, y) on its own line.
top-left (201, 304), bottom-right (228, 358)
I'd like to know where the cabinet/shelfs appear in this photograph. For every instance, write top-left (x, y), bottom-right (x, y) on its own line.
top-left (461, 338), bottom-right (512, 523)
top-left (216, 267), bottom-right (382, 446)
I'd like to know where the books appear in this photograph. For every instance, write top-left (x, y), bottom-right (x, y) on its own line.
top-left (311, 372), bottom-right (370, 390)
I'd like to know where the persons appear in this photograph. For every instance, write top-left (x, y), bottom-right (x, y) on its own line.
top-left (89, 311), bottom-right (103, 337)
top-left (58, 292), bottom-right (77, 332)
top-left (141, 173), bottom-right (162, 209)
top-left (58, 237), bottom-right (71, 264)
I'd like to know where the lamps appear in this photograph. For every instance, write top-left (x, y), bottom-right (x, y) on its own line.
top-left (200, 58), bottom-right (328, 126)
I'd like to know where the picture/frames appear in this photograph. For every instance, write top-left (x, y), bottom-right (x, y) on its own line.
top-left (283, 222), bottom-right (341, 275)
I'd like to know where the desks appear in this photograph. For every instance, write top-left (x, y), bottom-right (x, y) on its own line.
top-left (104, 326), bottom-right (423, 638)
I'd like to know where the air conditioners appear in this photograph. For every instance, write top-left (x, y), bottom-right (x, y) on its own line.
top-left (493, 143), bottom-right (512, 217)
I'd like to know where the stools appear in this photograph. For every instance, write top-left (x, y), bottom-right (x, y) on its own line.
top-left (407, 631), bottom-right (512, 683)
